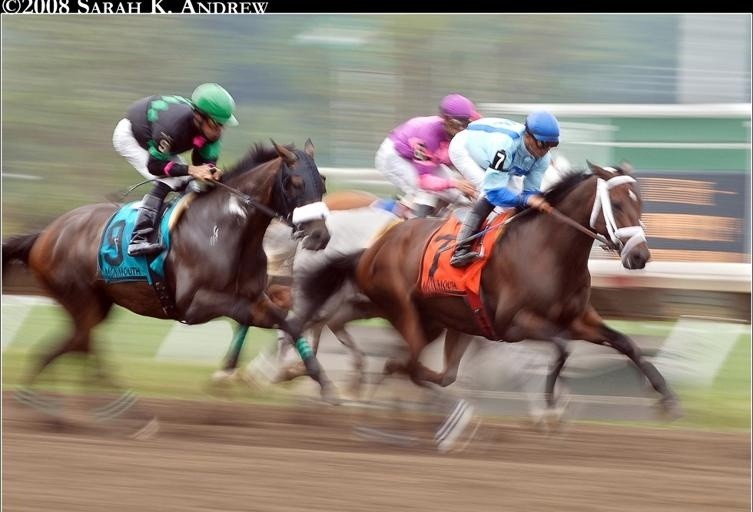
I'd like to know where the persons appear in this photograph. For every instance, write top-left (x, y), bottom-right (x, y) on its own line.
top-left (373, 93), bottom-right (481, 219)
top-left (112, 82), bottom-right (239, 256)
top-left (447, 108), bottom-right (560, 267)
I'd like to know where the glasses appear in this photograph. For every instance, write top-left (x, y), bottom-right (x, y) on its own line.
top-left (196, 109), bottom-right (225, 131)
top-left (447, 119), bottom-right (472, 128)
top-left (527, 131), bottom-right (559, 149)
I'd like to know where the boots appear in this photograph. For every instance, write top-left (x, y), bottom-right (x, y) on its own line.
top-left (450, 212), bottom-right (480, 267)
top-left (127, 194), bottom-right (162, 256)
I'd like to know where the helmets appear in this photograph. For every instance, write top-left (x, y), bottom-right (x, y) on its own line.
top-left (526, 110), bottom-right (559, 142)
top-left (439, 94), bottom-right (480, 120)
top-left (191, 84), bottom-right (239, 126)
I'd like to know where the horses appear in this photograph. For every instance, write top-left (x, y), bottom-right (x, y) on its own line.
top-left (264, 160), bottom-right (570, 403)
top-left (298, 158), bottom-right (679, 424)
top-left (1, 137), bottom-right (341, 408)
